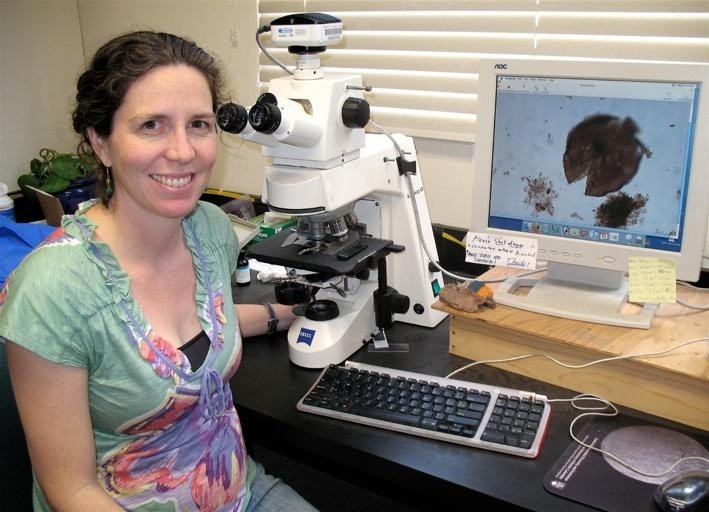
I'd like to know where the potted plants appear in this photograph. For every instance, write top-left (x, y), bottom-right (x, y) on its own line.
top-left (17, 148), bottom-right (107, 226)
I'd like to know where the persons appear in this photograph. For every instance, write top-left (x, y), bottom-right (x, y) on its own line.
top-left (0, 30), bottom-right (321, 512)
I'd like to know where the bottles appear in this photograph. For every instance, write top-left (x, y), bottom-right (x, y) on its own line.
top-left (235, 251), bottom-right (251, 285)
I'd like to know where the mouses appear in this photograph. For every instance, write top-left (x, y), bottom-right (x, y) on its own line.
top-left (653, 469), bottom-right (708, 511)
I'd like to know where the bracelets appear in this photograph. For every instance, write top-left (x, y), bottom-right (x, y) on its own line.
top-left (264, 302), bottom-right (278, 337)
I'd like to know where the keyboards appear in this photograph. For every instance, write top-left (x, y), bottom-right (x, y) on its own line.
top-left (296, 360), bottom-right (554, 459)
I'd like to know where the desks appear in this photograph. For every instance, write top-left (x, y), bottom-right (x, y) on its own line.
top-left (225, 219), bottom-right (709, 511)
top-left (432, 266), bottom-right (709, 433)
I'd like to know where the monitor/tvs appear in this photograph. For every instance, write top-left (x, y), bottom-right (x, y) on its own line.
top-left (472, 58), bottom-right (709, 329)
